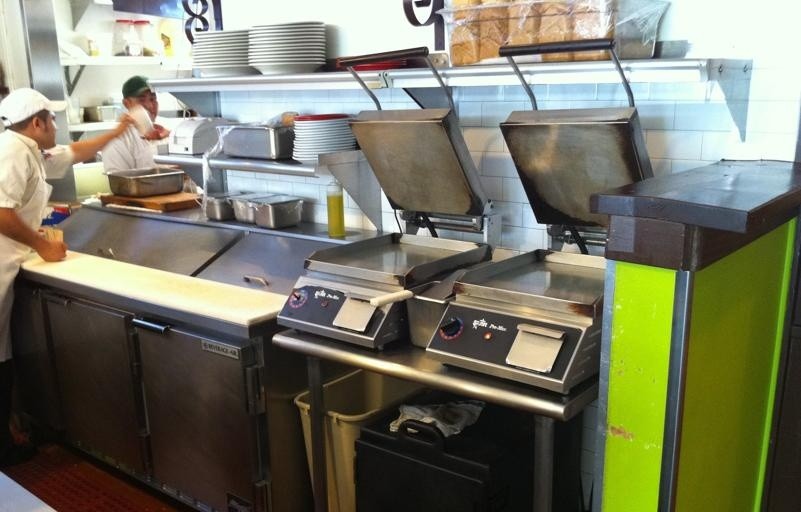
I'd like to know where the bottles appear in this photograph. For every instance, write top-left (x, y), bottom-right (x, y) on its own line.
top-left (112, 17), bottom-right (173, 57)
top-left (326, 176), bottom-right (346, 239)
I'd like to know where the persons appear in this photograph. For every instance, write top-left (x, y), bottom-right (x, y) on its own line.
top-left (102, 75), bottom-right (160, 175)
top-left (0, 87), bottom-right (132, 468)
top-left (140, 124), bottom-right (171, 141)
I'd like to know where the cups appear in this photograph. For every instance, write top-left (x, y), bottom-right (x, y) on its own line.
top-left (65, 96), bottom-right (80, 124)
top-left (88, 37), bottom-right (101, 56)
top-left (44, 223), bottom-right (64, 261)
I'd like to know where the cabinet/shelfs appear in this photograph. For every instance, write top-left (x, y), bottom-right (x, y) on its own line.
top-left (59, 54), bottom-right (194, 163)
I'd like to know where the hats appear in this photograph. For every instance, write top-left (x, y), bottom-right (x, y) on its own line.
top-left (123, 74), bottom-right (150, 98)
top-left (0, 88), bottom-right (68, 127)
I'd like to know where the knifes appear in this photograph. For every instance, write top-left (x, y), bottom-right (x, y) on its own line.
top-left (370, 282), bottom-right (434, 308)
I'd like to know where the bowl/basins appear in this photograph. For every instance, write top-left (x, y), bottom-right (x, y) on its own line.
top-left (70, 106), bottom-right (85, 123)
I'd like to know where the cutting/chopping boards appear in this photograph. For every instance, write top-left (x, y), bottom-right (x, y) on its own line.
top-left (100, 192), bottom-right (204, 213)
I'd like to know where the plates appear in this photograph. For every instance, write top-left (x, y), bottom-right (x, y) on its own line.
top-left (292, 113), bottom-right (358, 165)
top-left (247, 20), bottom-right (327, 75)
top-left (192, 28), bottom-right (250, 77)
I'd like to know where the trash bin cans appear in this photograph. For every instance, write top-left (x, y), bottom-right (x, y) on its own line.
top-left (292, 369), bottom-right (427, 512)
